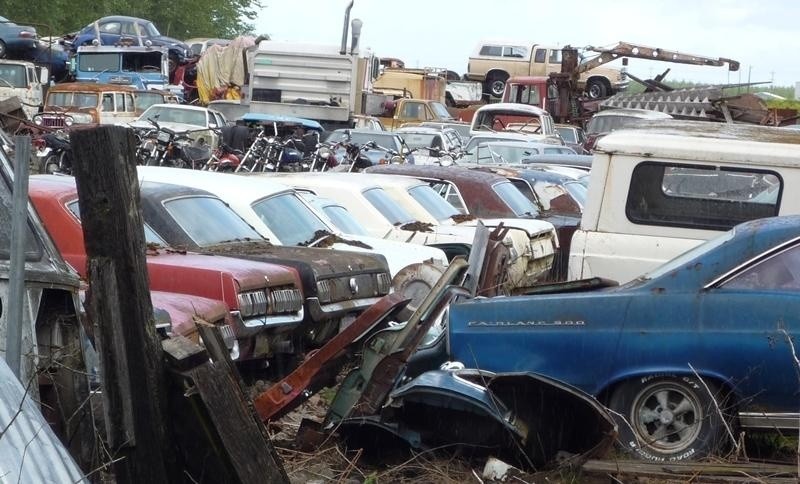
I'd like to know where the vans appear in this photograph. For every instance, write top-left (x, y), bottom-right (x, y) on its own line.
top-left (567, 119), bottom-right (800, 283)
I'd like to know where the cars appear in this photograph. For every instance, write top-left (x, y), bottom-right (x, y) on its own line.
top-left (445, 214), bottom-right (800, 466)
top-left (29, 172), bottom-right (396, 362)
top-left (1, 0), bottom-right (800, 326)
